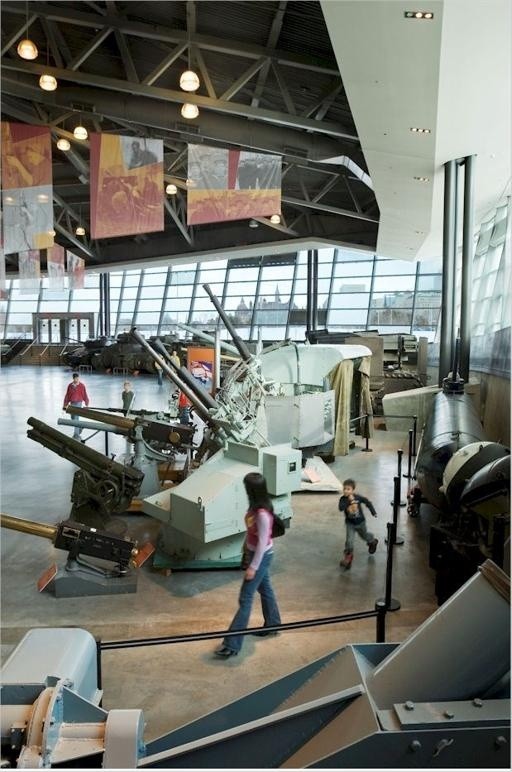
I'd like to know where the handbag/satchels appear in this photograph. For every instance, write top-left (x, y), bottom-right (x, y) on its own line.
top-left (271, 515), bottom-right (285, 537)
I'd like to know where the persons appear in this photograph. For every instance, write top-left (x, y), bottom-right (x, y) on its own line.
top-left (106, 180), bottom-right (130, 213)
top-left (50, 264), bottom-right (58, 280)
top-left (19, 202), bottom-right (35, 228)
top-left (337, 479), bottom-right (379, 568)
top-left (24, 250), bottom-right (37, 274)
top-left (68, 255), bottom-right (74, 282)
top-left (5, 146), bottom-right (52, 187)
top-left (16, 152), bottom-right (36, 187)
top-left (61, 372), bottom-right (90, 440)
top-left (179, 391), bottom-right (191, 425)
top-left (154, 352), bottom-right (165, 384)
top-left (190, 149), bottom-right (279, 222)
top-left (170, 351), bottom-right (180, 376)
top-left (127, 142), bottom-right (150, 170)
top-left (75, 260), bottom-right (81, 281)
top-left (215, 473), bottom-right (281, 660)
top-left (58, 265), bottom-right (64, 279)
top-left (122, 381), bottom-right (135, 416)
top-left (143, 176), bottom-right (160, 206)
top-left (121, 177), bottom-right (141, 201)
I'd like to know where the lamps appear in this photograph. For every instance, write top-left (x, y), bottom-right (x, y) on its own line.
top-left (181, 103), bottom-right (200, 120)
top-left (75, 205), bottom-right (85, 236)
top-left (248, 219), bottom-right (257, 227)
top-left (166, 183), bottom-right (178, 195)
top-left (17, 1), bottom-right (38, 60)
top-left (73, 116), bottom-right (89, 140)
top-left (57, 138), bottom-right (71, 151)
top-left (178, 33), bottom-right (200, 93)
top-left (270, 213), bottom-right (280, 225)
top-left (40, 39), bottom-right (57, 92)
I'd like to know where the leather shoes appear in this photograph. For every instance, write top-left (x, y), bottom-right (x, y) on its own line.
top-left (215, 647), bottom-right (237, 657)
top-left (254, 628), bottom-right (278, 636)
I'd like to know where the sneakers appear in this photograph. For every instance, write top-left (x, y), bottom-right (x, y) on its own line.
top-left (367, 539), bottom-right (378, 554)
top-left (340, 556), bottom-right (352, 568)
